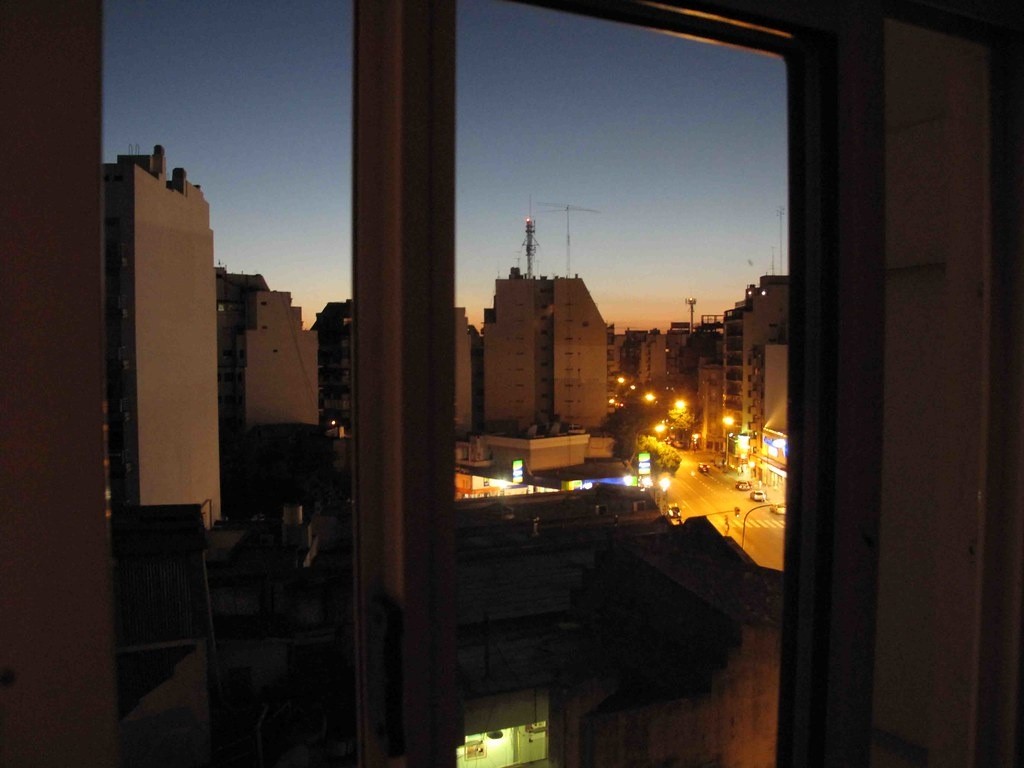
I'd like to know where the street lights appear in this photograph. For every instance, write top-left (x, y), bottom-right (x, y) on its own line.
top-left (635, 425), bottom-right (665, 453)
top-left (724, 417), bottom-right (734, 469)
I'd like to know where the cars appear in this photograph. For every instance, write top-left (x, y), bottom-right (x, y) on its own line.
top-left (698, 462), bottom-right (709, 474)
top-left (769, 503), bottom-right (786, 515)
top-left (736, 481), bottom-right (751, 491)
top-left (750, 491), bottom-right (766, 502)
top-left (669, 507), bottom-right (681, 518)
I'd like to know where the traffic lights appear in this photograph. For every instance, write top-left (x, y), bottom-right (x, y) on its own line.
top-left (734, 507), bottom-right (740, 517)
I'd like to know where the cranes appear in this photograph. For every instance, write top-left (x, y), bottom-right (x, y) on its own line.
top-left (538, 201), bottom-right (602, 278)
